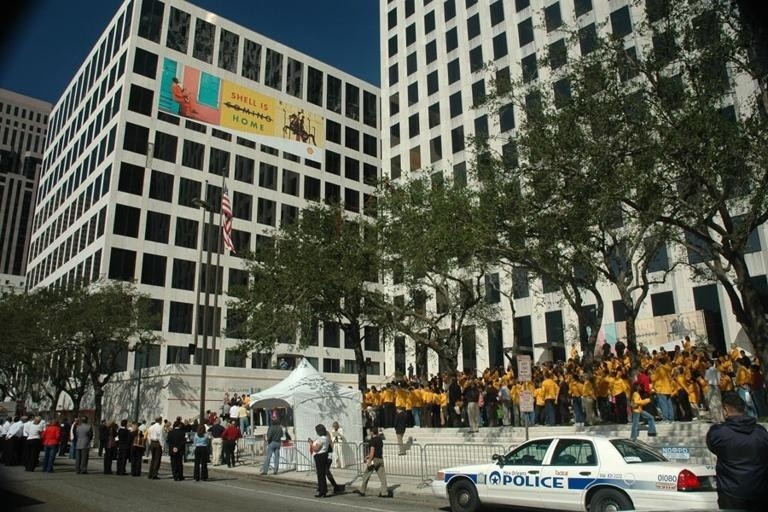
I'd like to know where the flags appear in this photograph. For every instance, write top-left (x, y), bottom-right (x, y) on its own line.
top-left (217, 178), bottom-right (257, 254)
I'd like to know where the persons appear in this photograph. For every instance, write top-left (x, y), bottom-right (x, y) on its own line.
top-left (362, 334), bottom-right (768, 440)
top-left (0, 389), bottom-right (288, 483)
top-left (307, 424), bottom-right (331, 498)
top-left (325, 430), bottom-right (343, 493)
top-left (172, 77), bottom-right (197, 117)
top-left (328, 421), bottom-right (346, 468)
top-left (355, 426), bottom-right (391, 498)
top-left (706, 392), bottom-right (768, 511)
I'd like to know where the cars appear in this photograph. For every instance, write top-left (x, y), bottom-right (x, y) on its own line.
top-left (429, 434), bottom-right (720, 512)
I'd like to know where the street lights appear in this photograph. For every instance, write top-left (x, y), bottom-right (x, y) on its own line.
top-left (190, 195), bottom-right (214, 433)
